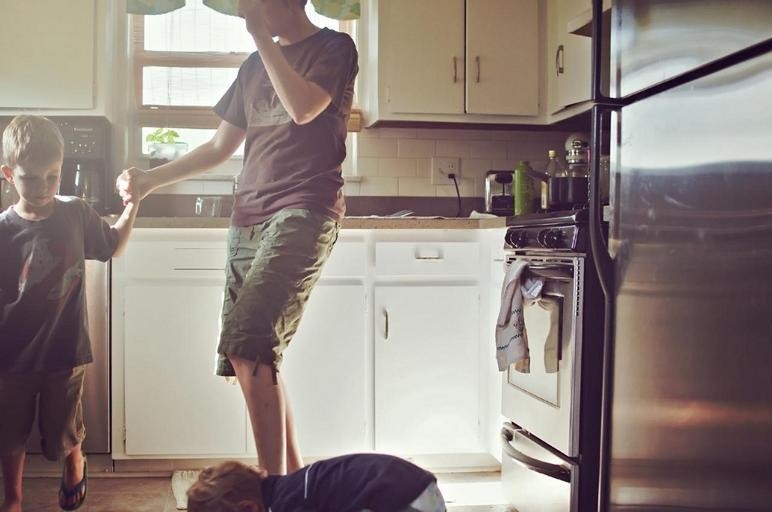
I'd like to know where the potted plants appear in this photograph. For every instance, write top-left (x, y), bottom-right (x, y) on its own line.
top-left (142, 126), bottom-right (190, 170)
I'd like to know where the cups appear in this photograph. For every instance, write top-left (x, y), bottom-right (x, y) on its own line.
top-left (195, 197), bottom-right (222, 217)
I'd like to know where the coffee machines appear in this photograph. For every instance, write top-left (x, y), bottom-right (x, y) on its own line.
top-left (1, 115), bottom-right (111, 219)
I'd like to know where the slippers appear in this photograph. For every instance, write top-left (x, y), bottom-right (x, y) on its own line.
top-left (59, 451), bottom-right (88, 511)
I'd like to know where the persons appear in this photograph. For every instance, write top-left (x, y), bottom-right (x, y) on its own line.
top-left (116, 1), bottom-right (359, 476)
top-left (0, 115), bottom-right (142, 512)
top-left (183, 456), bottom-right (446, 512)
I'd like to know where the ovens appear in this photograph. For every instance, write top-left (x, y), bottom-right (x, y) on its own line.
top-left (499, 252), bottom-right (605, 512)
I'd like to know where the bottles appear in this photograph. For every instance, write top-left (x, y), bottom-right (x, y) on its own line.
top-left (515, 147), bottom-right (589, 215)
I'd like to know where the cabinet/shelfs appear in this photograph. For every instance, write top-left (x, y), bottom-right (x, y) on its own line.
top-left (356, 0), bottom-right (594, 131)
top-left (112, 227), bottom-right (370, 476)
top-left (370, 230), bottom-right (502, 476)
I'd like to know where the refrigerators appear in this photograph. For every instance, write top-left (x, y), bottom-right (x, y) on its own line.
top-left (589, 1), bottom-right (772, 510)
